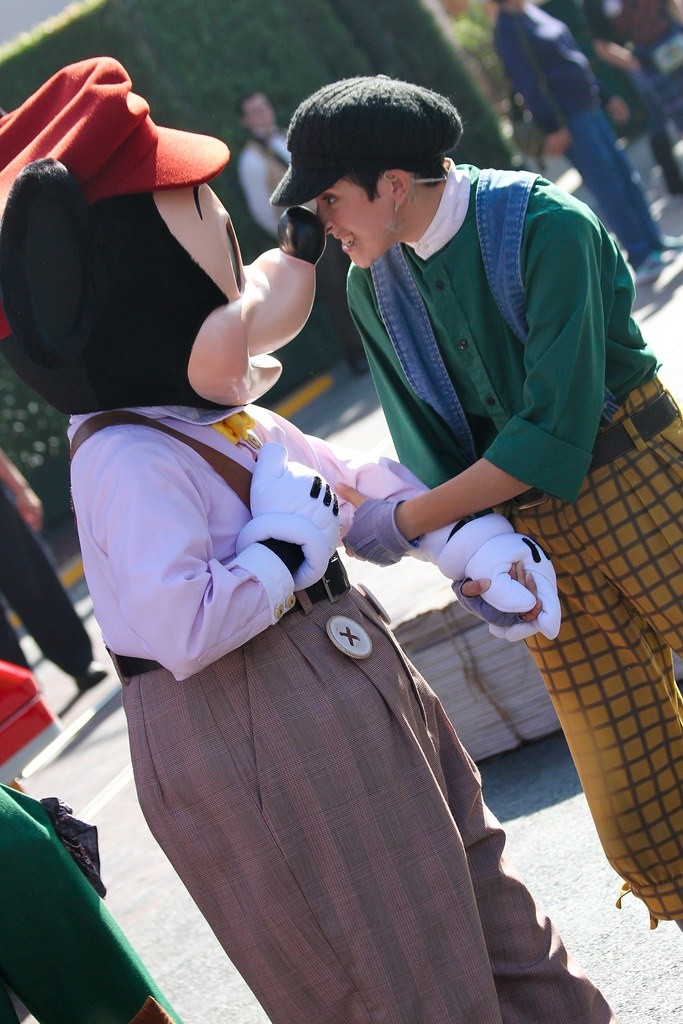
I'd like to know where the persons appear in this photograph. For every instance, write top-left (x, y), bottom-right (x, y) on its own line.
top-left (269, 74), bottom-right (683, 929)
top-left (0, 786), bottom-right (183, 1024)
top-left (0, 449), bottom-right (109, 689)
top-left (441, 0), bottom-right (683, 285)
top-left (234, 89), bottom-right (368, 375)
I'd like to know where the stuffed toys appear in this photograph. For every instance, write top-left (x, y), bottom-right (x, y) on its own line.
top-left (0, 57), bottom-right (615, 1024)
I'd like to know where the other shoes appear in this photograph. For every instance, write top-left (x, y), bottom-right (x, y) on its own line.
top-left (353, 360), bottom-right (370, 374)
top-left (635, 236), bottom-right (683, 283)
top-left (78, 667), bottom-right (107, 691)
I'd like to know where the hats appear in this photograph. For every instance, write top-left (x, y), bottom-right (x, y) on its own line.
top-left (270, 74), bottom-right (462, 207)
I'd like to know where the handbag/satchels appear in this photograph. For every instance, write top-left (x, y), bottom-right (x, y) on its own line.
top-left (509, 88), bottom-right (547, 157)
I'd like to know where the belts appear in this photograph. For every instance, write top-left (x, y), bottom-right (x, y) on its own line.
top-left (513, 392), bottom-right (678, 509)
